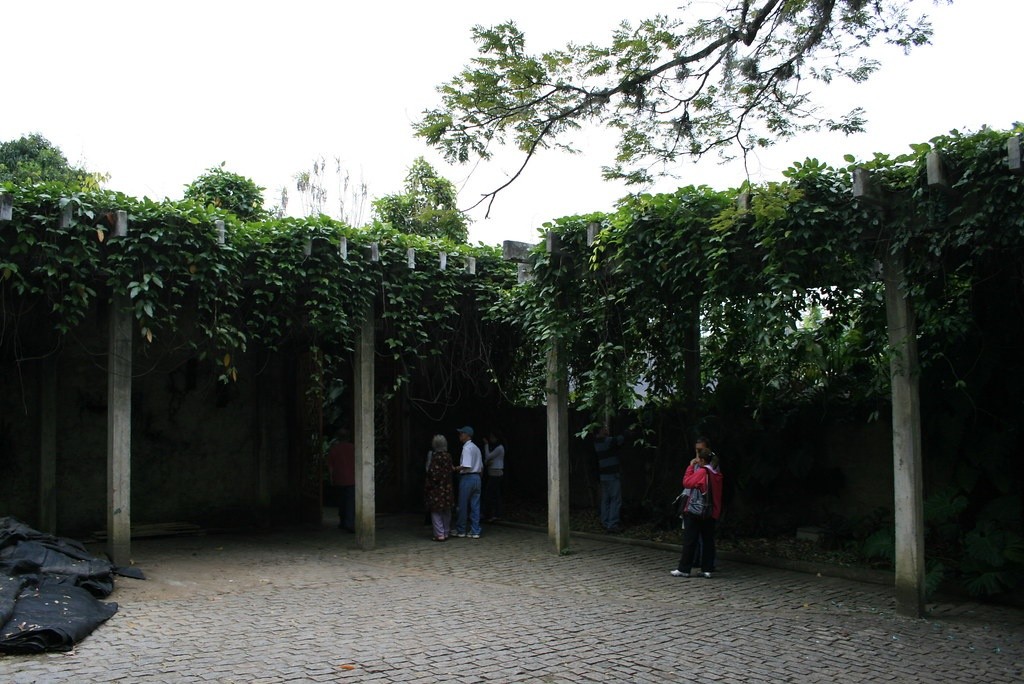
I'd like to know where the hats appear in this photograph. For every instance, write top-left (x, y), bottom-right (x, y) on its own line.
top-left (457, 427), bottom-right (473, 437)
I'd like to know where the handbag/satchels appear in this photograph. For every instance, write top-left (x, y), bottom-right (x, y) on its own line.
top-left (687, 489), bottom-right (713, 521)
top-left (673, 488), bottom-right (691, 514)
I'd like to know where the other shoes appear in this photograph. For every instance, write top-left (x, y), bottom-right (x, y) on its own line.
top-left (696, 570), bottom-right (712, 578)
top-left (488, 517), bottom-right (502, 523)
top-left (600, 522), bottom-right (624, 534)
top-left (670, 569), bottom-right (690, 577)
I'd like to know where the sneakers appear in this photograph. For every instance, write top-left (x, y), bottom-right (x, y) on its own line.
top-left (449, 529), bottom-right (466, 538)
top-left (466, 529), bottom-right (480, 539)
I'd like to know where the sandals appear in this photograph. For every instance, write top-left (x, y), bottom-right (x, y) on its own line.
top-left (431, 536), bottom-right (449, 542)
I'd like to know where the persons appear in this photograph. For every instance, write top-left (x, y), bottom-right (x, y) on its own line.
top-left (450, 426), bottom-right (484, 538)
top-left (327, 429), bottom-right (355, 532)
top-left (423, 434), bottom-right (455, 541)
top-left (670, 437), bottom-right (723, 579)
top-left (483, 429), bottom-right (509, 522)
top-left (593, 425), bottom-right (631, 532)
